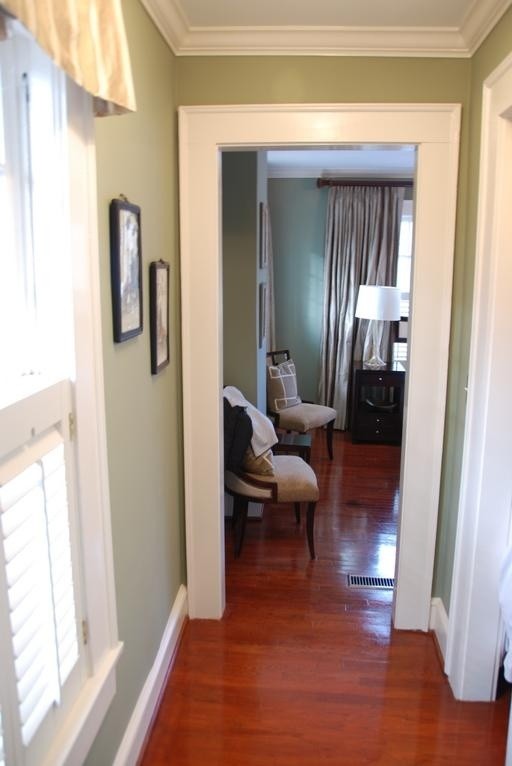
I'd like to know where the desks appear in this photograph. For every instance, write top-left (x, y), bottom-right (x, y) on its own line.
top-left (273, 435), bottom-right (317, 463)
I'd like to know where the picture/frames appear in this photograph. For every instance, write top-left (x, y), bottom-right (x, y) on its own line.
top-left (110, 198), bottom-right (143, 343)
top-left (262, 200), bottom-right (271, 268)
top-left (260, 281), bottom-right (268, 348)
top-left (150, 262), bottom-right (171, 373)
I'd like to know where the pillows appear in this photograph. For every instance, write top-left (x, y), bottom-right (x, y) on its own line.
top-left (267, 359), bottom-right (302, 408)
top-left (242, 412), bottom-right (275, 478)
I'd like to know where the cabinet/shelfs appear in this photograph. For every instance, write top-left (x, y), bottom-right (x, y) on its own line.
top-left (350, 361), bottom-right (405, 444)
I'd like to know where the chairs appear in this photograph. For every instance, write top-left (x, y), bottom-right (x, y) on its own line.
top-left (267, 351), bottom-right (335, 461)
top-left (225, 386), bottom-right (320, 559)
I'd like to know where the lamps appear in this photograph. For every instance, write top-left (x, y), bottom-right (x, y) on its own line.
top-left (355, 284), bottom-right (401, 365)
